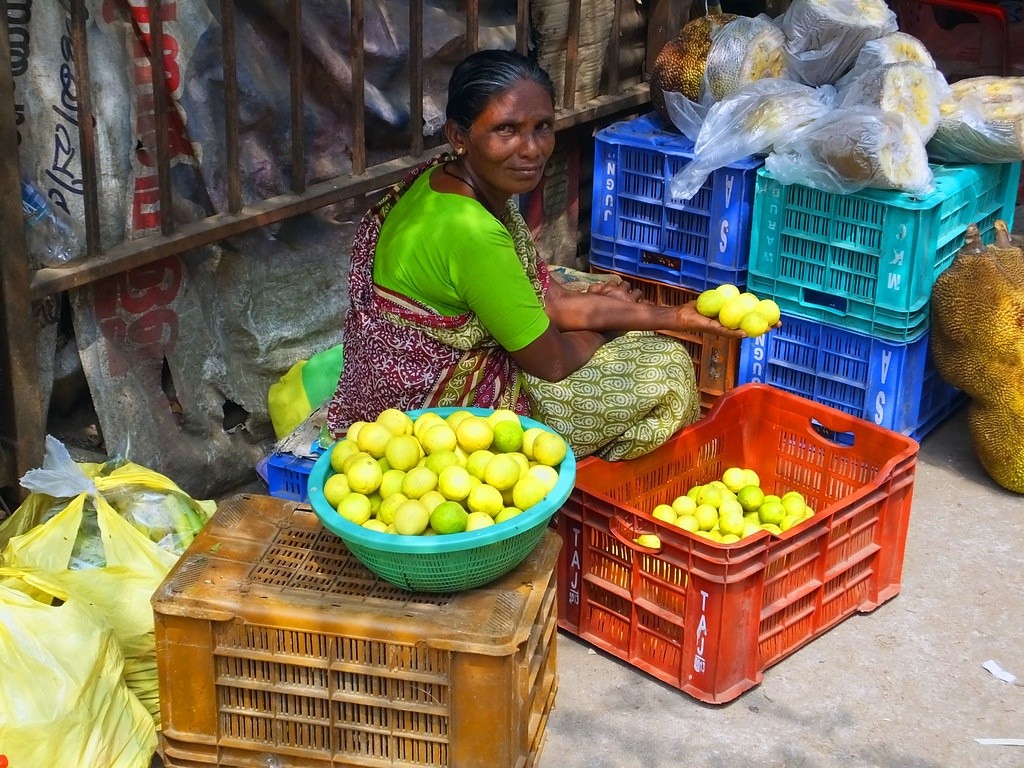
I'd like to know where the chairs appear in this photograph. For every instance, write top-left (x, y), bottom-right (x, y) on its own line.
top-left (895, 0), bottom-right (1010, 77)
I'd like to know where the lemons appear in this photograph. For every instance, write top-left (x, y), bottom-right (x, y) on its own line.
top-left (631, 465), bottom-right (815, 550)
top-left (322, 407), bottom-right (566, 536)
top-left (696, 283), bottom-right (781, 338)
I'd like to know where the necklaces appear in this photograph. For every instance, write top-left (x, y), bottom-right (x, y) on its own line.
top-left (443, 160), bottom-right (499, 220)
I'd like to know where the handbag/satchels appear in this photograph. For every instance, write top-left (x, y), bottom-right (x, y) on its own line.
top-left (655, 0), bottom-right (1024, 200)
top-left (0, 433), bottom-right (220, 768)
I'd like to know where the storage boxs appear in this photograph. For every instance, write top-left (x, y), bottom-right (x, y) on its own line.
top-left (748, 161), bottom-right (1022, 343)
top-left (267, 400), bottom-right (337, 502)
top-left (148, 493), bottom-right (559, 768)
top-left (553, 383), bottom-right (921, 705)
top-left (736, 315), bottom-right (968, 443)
top-left (586, 108), bottom-right (765, 293)
top-left (588, 264), bottom-right (737, 421)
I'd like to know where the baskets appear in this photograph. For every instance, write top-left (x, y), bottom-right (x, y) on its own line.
top-left (307, 405), bottom-right (577, 593)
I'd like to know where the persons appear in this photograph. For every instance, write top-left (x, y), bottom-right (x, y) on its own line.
top-left (326, 49), bottom-right (783, 462)
